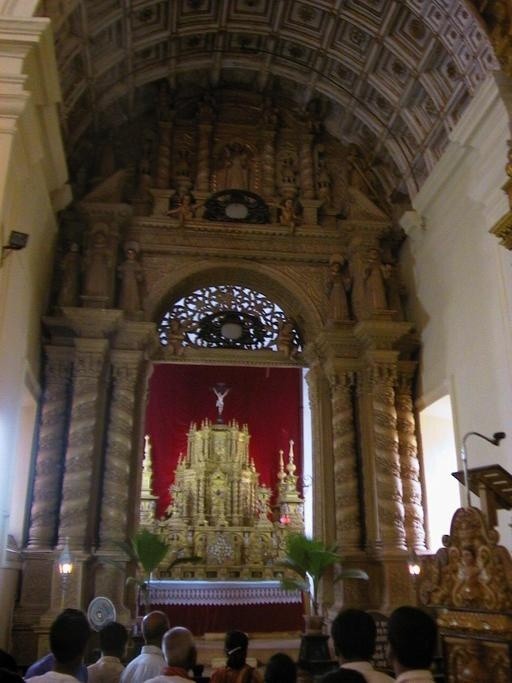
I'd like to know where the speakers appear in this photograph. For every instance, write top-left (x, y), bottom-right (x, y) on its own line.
top-left (11, 230), bottom-right (28, 248)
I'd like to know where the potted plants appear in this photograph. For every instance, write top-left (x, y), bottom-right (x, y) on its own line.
top-left (270, 531), bottom-right (369, 637)
top-left (97, 525), bottom-right (203, 637)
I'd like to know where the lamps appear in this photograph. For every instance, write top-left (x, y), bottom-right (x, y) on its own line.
top-left (406, 546), bottom-right (422, 606)
top-left (3, 230), bottom-right (29, 250)
top-left (56, 535), bottom-right (74, 615)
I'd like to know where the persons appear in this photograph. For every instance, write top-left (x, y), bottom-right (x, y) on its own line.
top-left (24, 604), bottom-right (446, 683)
top-left (329, 247), bottom-right (406, 323)
top-left (213, 387), bottom-right (231, 415)
top-left (122, 136), bottom-right (369, 224)
top-left (455, 546), bottom-right (480, 600)
top-left (60, 230), bottom-right (145, 313)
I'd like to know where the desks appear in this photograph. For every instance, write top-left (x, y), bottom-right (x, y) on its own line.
top-left (137, 579), bottom-right (307, 638)
